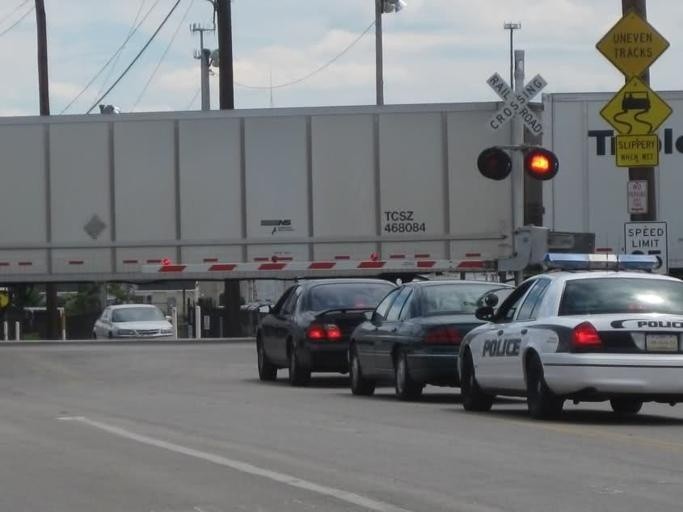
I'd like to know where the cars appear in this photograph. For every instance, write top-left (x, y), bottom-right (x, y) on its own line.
top-left (91, 303), bottom-right (174, 340)
top-left (453, 269), bottom-right (682, 417)
top-left (254, 278), bottom-right (397, 387)
top-left (345, 279), bottom-right (517, 399)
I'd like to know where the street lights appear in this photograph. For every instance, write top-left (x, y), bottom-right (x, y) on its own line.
top-left (500, 21), bottom-right (520, 93)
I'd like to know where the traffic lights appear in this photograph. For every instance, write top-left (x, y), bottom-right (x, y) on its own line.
top-left (478, 147), bottom-right (556, 179)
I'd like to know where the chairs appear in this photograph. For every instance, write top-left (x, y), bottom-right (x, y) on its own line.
top-left (439, 295), bottom-right (461, 312)
